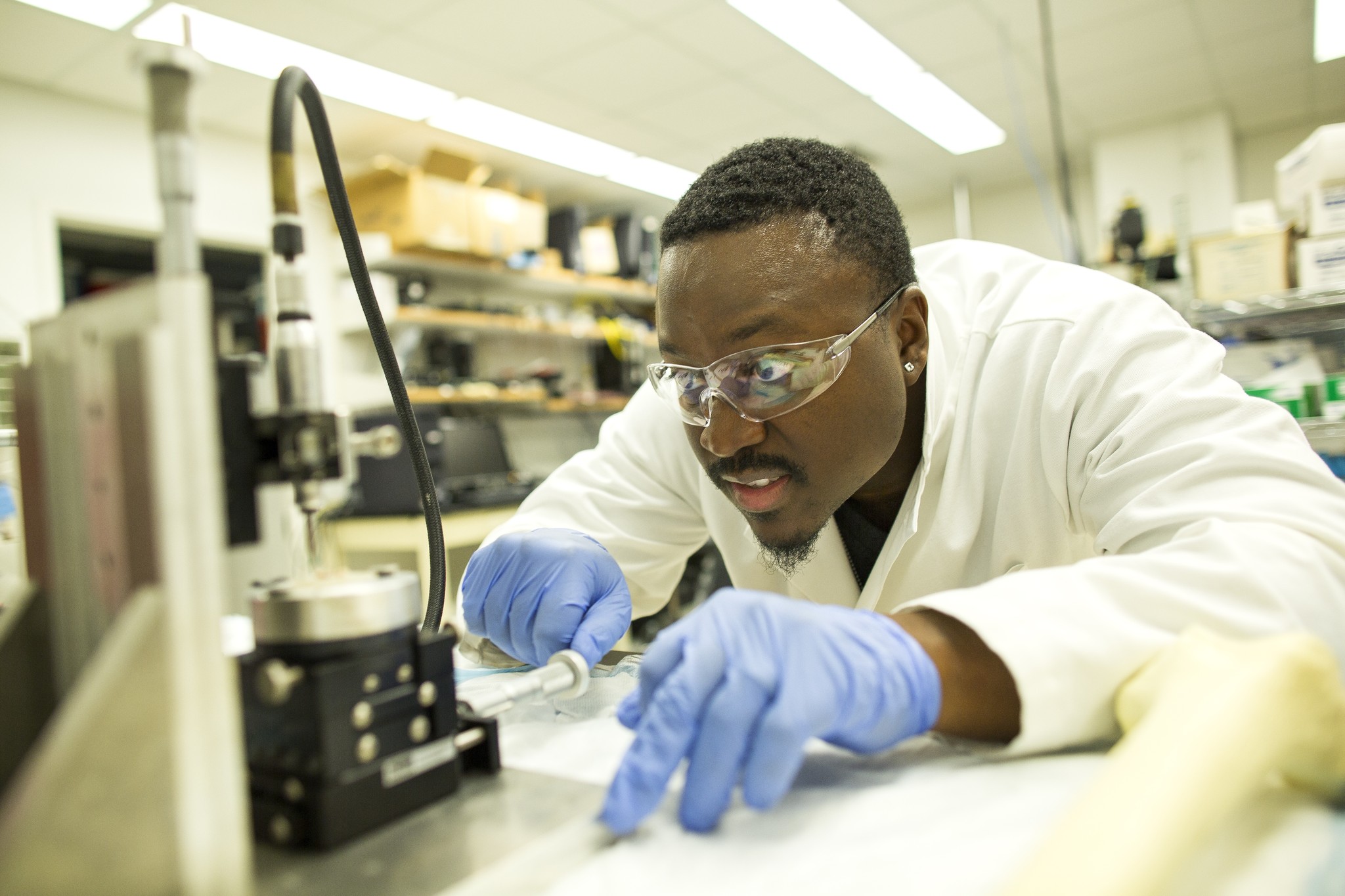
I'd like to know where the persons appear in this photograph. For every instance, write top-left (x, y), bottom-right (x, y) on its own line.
top-left (453, 139), bottom-right (1345, 836)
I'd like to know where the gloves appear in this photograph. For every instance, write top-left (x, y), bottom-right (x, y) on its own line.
top-left (463, 530), bottom-right (632, 669)
top-left (597, 585), bottom-right (941, 837)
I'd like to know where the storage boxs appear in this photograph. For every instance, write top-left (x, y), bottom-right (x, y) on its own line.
top-left (1189, 226), bottom-right (1292, 307)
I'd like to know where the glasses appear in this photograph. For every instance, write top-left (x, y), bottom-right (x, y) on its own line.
top-left (646, 281), bottom-right (919, 427)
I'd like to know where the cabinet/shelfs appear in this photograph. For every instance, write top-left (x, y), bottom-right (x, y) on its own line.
top-left (329, 248), bottom-right (655, 419)
